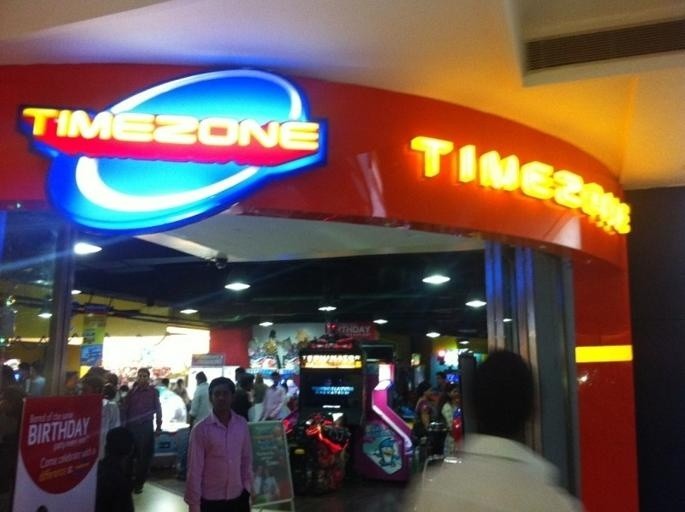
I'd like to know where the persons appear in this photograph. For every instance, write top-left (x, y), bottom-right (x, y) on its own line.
top-left (0, 359), bottom-right (293, 512)
top-left (436, 384), bottom-right (460, 456)
top-left (396, 349), bottom-right (586, 511)
top-left (412, 380), bottom-right (443, 456)
top-left (435, 372), bottom-right (451, 405)
